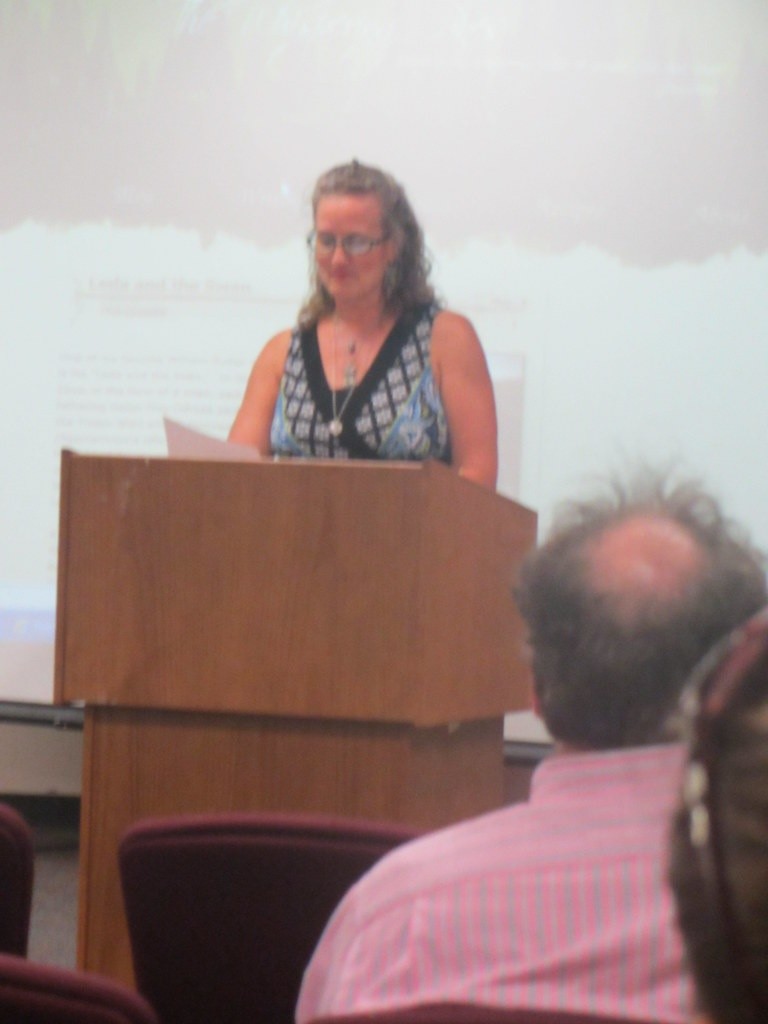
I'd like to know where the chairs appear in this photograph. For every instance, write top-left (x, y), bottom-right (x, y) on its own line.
top-left (0, 801), bottom-right (412, 1024)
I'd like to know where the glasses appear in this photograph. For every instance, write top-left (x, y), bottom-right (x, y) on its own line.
top-left (670, 609), bottom-right (768, 1023)
top-left (313, 232), bottom-right (390, 257)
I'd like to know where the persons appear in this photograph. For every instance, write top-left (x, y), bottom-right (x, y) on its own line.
top-left (660, 603), bottom-right (768, 1023)
top-left (224, 153), bottom-right (499, 494)
top-left (291, 464), bottom-right (767, 1023)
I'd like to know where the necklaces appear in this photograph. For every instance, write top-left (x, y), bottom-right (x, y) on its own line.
top-left (329, 313), bottom-right (383, 438)
